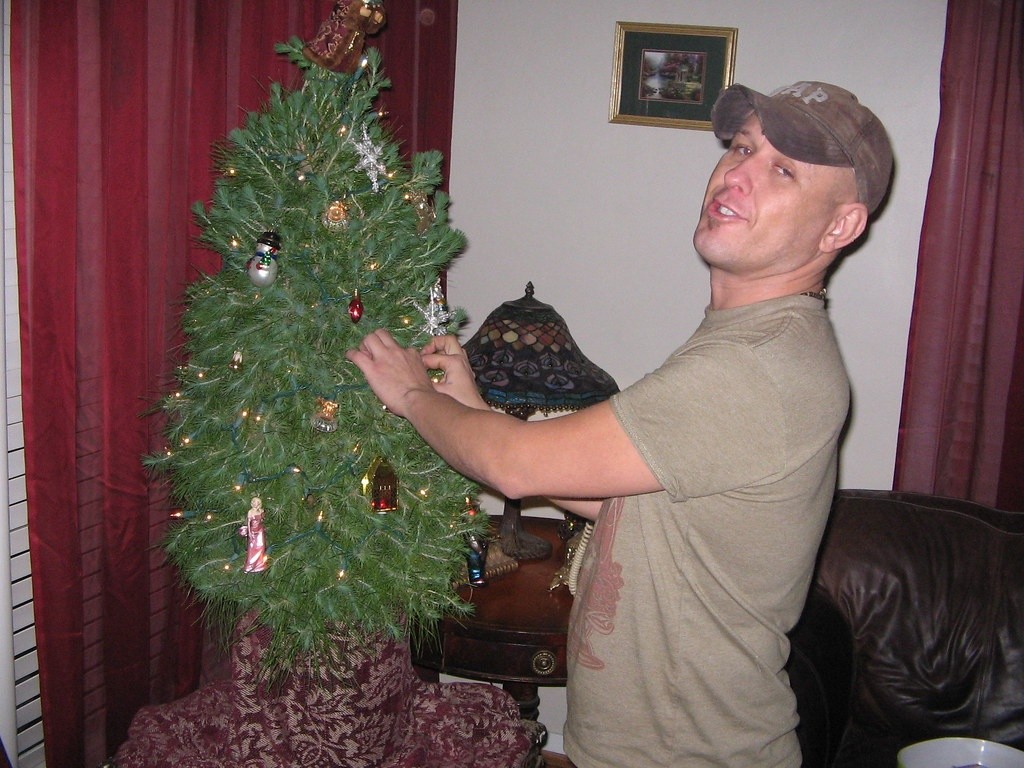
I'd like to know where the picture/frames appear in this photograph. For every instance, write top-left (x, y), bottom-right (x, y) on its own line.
top-left (607, 20), bottom-right (739, 132)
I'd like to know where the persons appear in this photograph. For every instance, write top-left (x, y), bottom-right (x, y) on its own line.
top-left (345, 81), bottom-right (894, 768)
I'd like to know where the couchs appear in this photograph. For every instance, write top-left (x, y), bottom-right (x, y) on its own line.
top-left (785, 489), bottom-right (1022, 768)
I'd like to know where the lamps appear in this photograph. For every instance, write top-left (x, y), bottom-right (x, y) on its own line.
top-left (460, 282), bottom-right (620, 561)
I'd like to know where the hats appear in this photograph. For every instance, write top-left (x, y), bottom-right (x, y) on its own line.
top-left (710, 80), bottom-right (893, 217)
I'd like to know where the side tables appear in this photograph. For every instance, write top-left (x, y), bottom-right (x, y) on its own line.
top-left (412, 514), bottom-right (590, 724)
